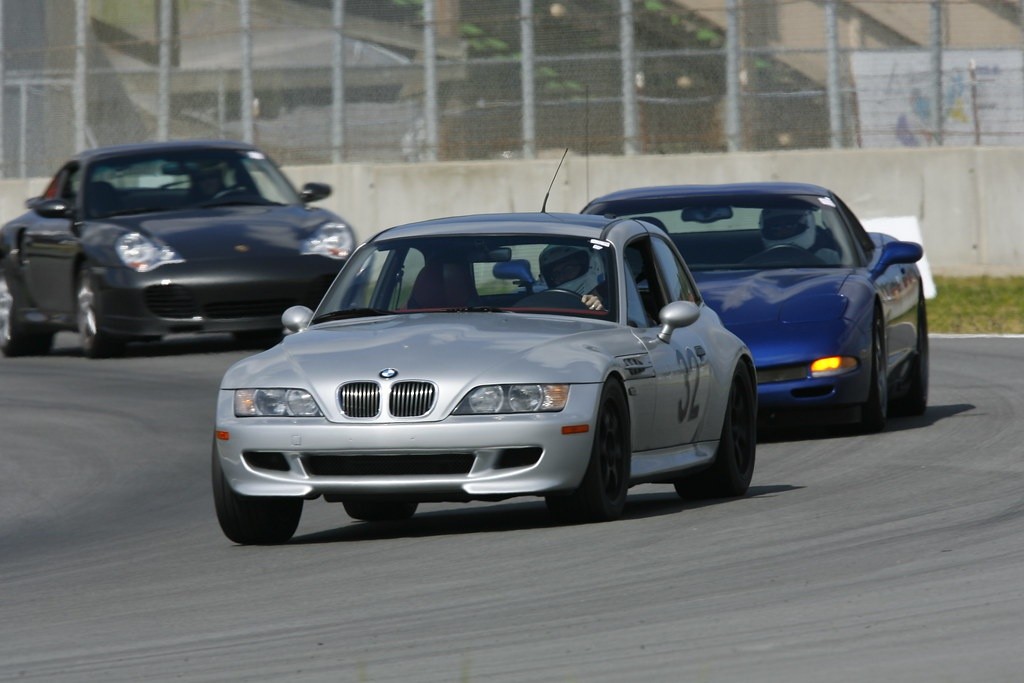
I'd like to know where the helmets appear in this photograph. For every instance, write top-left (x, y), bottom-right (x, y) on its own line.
top-left (190, 159), bottom-right (229, 196)
top-left (539, 245), bottom-right (606, 296)
top-left (759, 208), bottom-right (817, 250)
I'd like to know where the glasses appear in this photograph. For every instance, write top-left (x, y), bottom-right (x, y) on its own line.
top-left (550, 264), bottom-right (580, 280)
top-left (768, 222), bottom-right (798, 231)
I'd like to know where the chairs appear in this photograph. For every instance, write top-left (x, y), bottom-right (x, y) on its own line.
top-left (85, 181), bottom-right (125, 216)
top-left (407, 259), bottom-right (481, 308)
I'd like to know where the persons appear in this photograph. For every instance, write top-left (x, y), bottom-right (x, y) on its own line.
top-left (192, 170), bottom-right (225, 203)
top-left (539, 243), bottom-right (609, 312)
top-left (759, 207), bottom-right (840, 266)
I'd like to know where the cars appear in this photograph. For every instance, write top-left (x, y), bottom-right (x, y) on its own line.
top-left (0, 143), bottom-right (355, 358)
top-left (581, 180), bottom-right (927, 438)
top-left (214, 212), bottom-right (760, 545)
